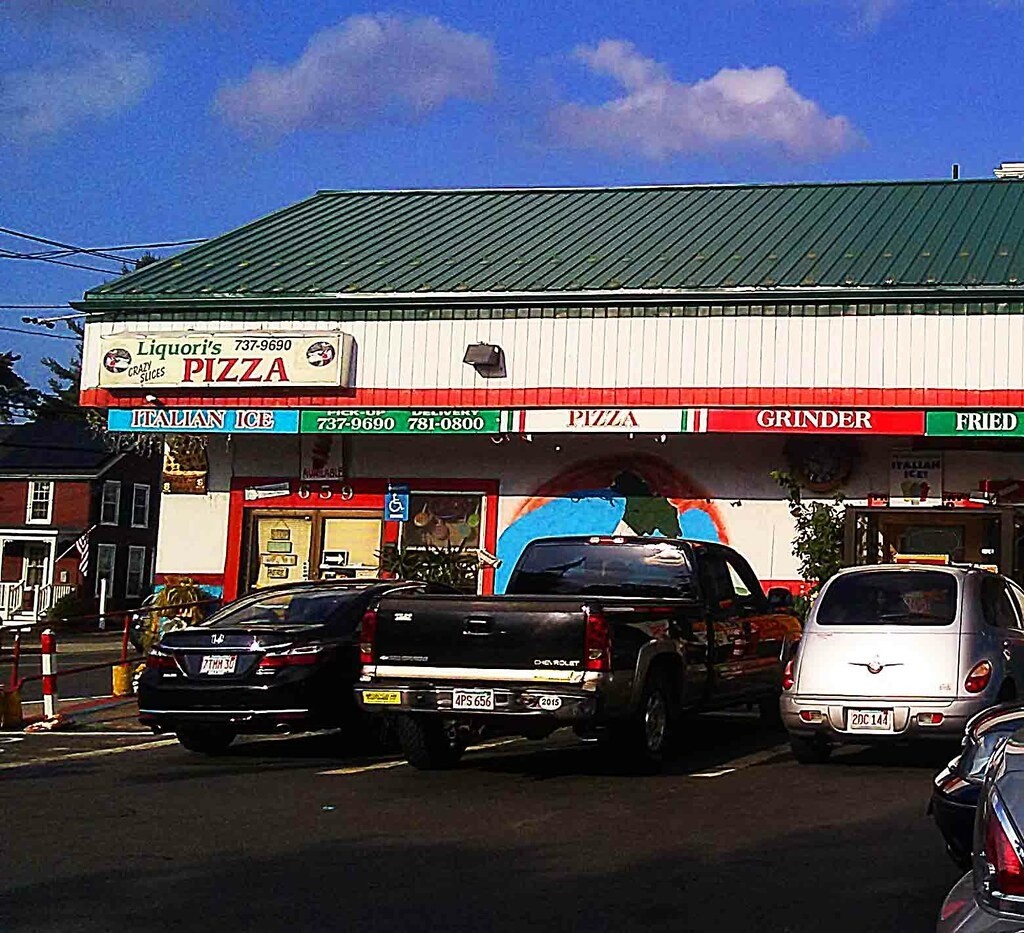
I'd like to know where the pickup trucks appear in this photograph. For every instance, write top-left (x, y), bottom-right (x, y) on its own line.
top-left (352, 533), bottom-right (806, 776)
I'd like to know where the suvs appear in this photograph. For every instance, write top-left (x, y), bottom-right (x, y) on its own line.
top-left (778, 560), bottom-right (1024, 767)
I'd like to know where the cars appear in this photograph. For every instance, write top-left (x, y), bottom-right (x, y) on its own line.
top-left (924, 696), bottom-right (1024, 871)
top-left (134, 580), bottom-right (463, 756)
top-left (938, 726), bottom-right (1024, 933)
